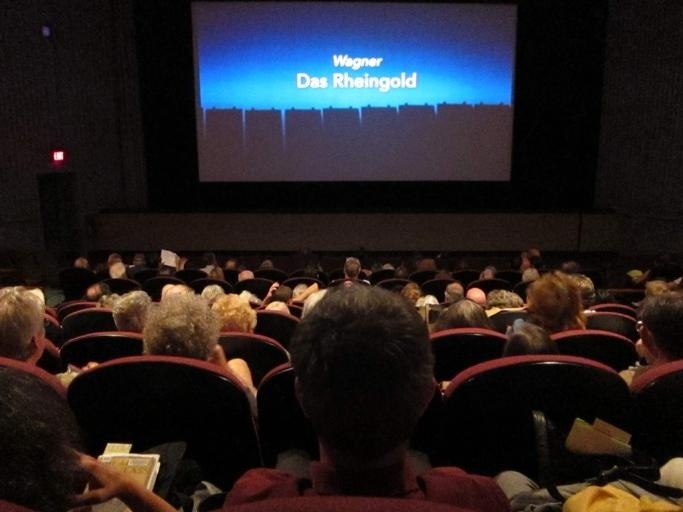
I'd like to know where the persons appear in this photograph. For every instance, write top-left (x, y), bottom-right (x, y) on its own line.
top-left (1, 251), bottom-right (682, 512)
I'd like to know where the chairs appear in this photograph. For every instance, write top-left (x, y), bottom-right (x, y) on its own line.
top-left (0, 357), bottom-right (73, 512)
top-left (187, 277), bottom-right (235, 297)
top-left (285, 276), bottom-right (321, 289)
top-left (514, 277), bottom-right (533, 296)
top-left (233, 277), bottom-right (276, 297)
top-left (632, 360), bottom-right (683, 472)
top-left (133, 267), bottom-right (160, 277)
top-left (409, 270), bottom-right (437, 283)
top-left (60, 332), bottom-right (148, 367)
top-left (96, 276), bottom-right (143, 295)
top-left (62, 308), bottom-right (118, 340)
top-left (36, 337), bottom-right (58, 370)
top-left (330, 268), bottom-right (365, 279)
top-left (253, 268), bottom-right (288, 280)
top-left (549, 329), bottom-right (637, 372)
top-left (256, 359), bottom-right (443, 489)
top-left (420, 278), bottom-right (458, 302)
top-left (367, 269), bottom-right (392, 282)
top-left (44, 299), bottom-right (100, 316)
top-left (593, 303), bottom-right (638, 316)
top-left (255, 309), bottom-right (300, 349)
top-left (441, 356), bottom-right (631, 489)
top-left (44, 313), bottom-right (59, 343)
top-left (466, 278), bottom-right (513, 296)
top-left (221, 267), bottom-right (240, 283)
top-left (66, 354), bottom-right (256, 511)
top-left (494, 269), bottom-right (521, 279)
top-left (293, 268), bottom-right (328, 281)
top-left (216, 330), bottom-right (291, 385)
top-left (452, 269), bottom-right (478, 281)
top-left (375, 278), bottom-right (413, 291)
top-left (430, 326), bottom-right (509, 380)
top-left (247, 299), bottom-right (304, 315)
top-left (173, 269), bottom-right (206, 279)
top-left (143, 276), bottom-right (187, 299)
top-left (587, 311), bottom-right (639, 338)
